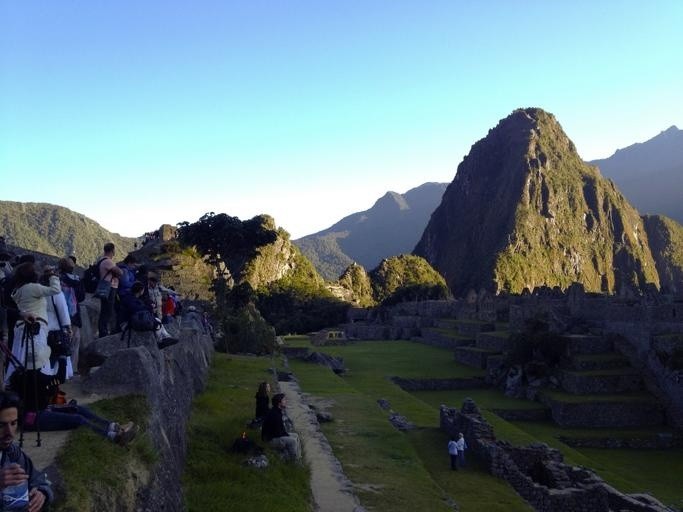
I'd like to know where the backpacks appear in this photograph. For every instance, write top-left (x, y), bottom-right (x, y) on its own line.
top-left (93, 269), bottom-right (113, 299)
top-left (131, 310), bottom-right (161, 331)
top-left (83, 258), bottom-right (107, 293)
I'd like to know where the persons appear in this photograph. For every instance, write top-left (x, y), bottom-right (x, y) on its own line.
top-left (454, 431), bottom-right (467, 468)
top-left (259, 393), bottom-right (302, 465)
top-left (0, 396), bottom-right (55, 511)
top-left (184, 305), bottom-right (199, 313)
top-left (447, 435), bottom-right (458, 470)
top-left (201, 311), bottom-right (213, 337)
top-left (254, 381), bottom-right (273, 420)
top-left (0, 235), bottom-right (183, 445)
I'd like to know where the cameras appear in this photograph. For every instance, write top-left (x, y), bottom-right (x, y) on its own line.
top-left (28, 321), bottom-right (41, 335)
top-left (44, 264), bottom-right (56, 273)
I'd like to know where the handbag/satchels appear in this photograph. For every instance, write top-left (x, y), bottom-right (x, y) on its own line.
top-left (0, 341), bottom-right (59, 410)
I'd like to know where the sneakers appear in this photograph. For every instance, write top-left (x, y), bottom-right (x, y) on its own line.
top-left (113, 421), bottom-right (139, 448)
top-left (158, 337), bottom-right (179, 349)
